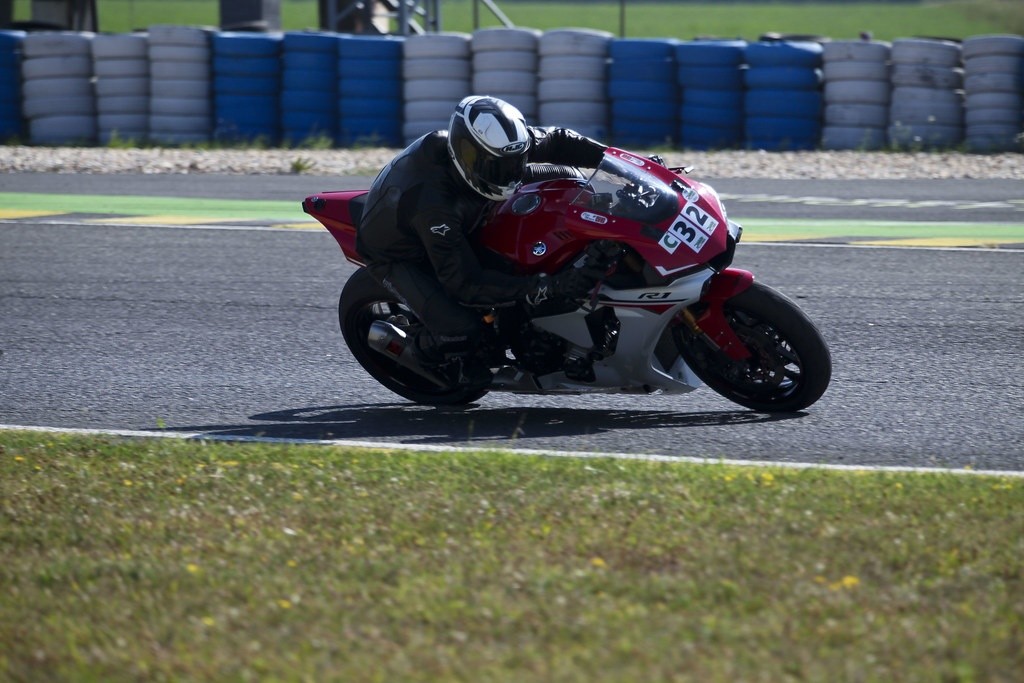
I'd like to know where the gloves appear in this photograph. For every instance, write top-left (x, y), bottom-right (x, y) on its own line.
top-left (618, 155), bottom-right (668, 189)
top-left (524, 268), bottom-right (599, 308)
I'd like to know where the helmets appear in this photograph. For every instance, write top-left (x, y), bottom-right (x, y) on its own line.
top-left (448, 96), bottom-right (530, 201)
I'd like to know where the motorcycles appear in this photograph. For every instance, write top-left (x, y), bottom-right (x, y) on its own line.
top-left (302, 146), bottom-right (832, 414)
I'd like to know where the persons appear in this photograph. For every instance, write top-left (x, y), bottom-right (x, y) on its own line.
top-left (356, 94), bottom-right (666, 404)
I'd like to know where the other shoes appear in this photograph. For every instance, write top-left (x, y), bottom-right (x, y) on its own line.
top-left (411, 326), bottom-right (481, 390)
